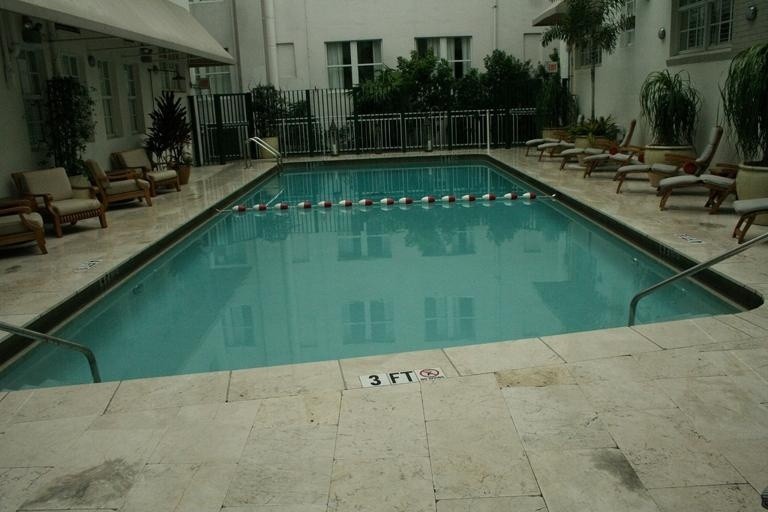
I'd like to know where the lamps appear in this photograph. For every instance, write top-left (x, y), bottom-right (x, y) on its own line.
top-left (149, 68), bottom-right (186, 81)
top-left (745, 5), bottom-right (757, 20)
top-left (658, 28), bottom-right (666, 39)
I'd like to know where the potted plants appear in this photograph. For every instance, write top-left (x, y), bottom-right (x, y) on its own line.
top-left (639, 70), bottom-right (707, 187)
top-left (720, 41), bottom-right (768, 226)
top-left (145, 91), bottom-right (195, 184)
top-left (249, 81), bottom-right (288, 158)
top-left (575, 114), bottom-right (622, 167)
top-left (537, 77), bottom-right (580, 152)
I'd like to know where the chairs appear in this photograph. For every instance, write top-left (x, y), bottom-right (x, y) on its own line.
top-left (85, 159), bottom-right (153, 207)
top-left (10, 166), bottom-right (108, 237)
top-left (0, 199), bottom-right (49, 255)
top-left (111, 148), bottom-right (181, 196)
top-left (526, 119), bottom-right (768, 243)
